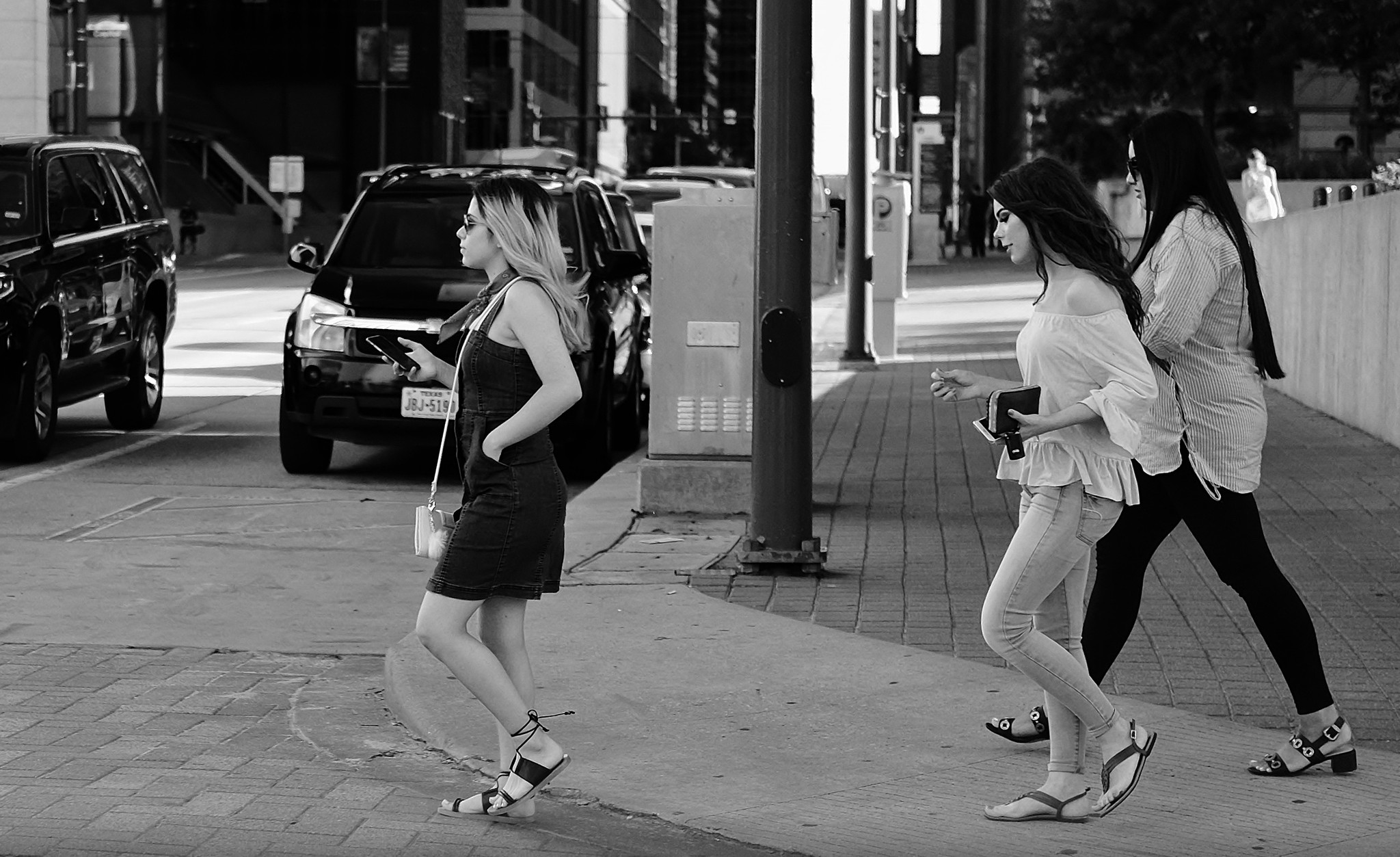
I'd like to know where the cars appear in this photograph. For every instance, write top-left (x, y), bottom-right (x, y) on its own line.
top-left (469, 147), bottom-right (757, 262)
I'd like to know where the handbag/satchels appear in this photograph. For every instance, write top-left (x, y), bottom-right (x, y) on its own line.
top-left (413, 504), bottom-right (456, 561)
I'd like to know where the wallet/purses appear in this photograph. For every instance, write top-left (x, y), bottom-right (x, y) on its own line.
top-left (986, 384), bottom-right (1041, 435)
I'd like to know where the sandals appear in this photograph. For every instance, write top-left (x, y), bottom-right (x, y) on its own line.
top-left (1092, 718), bottom-right (1157, 818)
top-left (437, 767), bottom-right (536, 824)
top-left (487, 705), bottom-right (576, 815)
top-left (983, 785), bottom-right (1092, 824)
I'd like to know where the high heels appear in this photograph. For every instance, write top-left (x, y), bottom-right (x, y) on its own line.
top-left (1247, 715), bottom-right (1358, 777)
top-left (985, 704), bottom-right (1049, 744)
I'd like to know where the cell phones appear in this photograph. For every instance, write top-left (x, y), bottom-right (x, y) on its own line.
top-left (972, 415), bottom-right (1020, 442)
top-left (365, 335), bottom-right (421, 371)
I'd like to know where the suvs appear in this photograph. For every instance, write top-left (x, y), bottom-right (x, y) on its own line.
top-left (0, 133), bottom-right (177, 454)
top-left (279, 171), bottom-right (653, 481)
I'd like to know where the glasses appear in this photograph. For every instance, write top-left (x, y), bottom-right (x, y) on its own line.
top-left (462, 213), bottom-right (486, 232)
top-left (1127, 157), bottom-right (1139, 179)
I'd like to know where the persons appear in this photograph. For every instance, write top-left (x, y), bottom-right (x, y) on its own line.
top-left (1239, 145), bottom-right (1286, 222)
top-left (177, 199), bottom-right (200, 254)
top-left (930, 156), bottom-right (1159, 823)
top-left (983, 111), bottom-right (1359, 779)
top-left (378, 173), bottom-right (584, 821)
